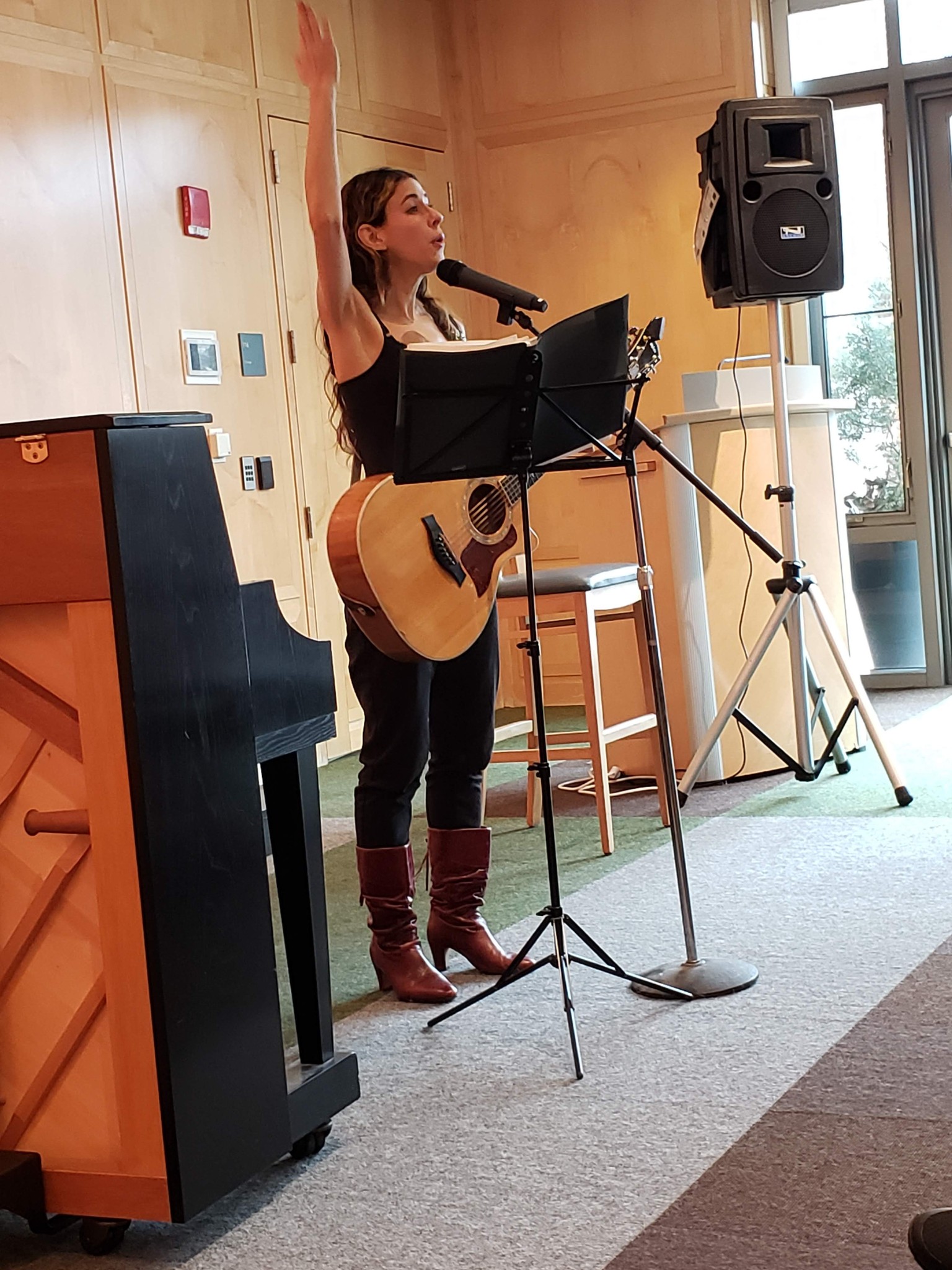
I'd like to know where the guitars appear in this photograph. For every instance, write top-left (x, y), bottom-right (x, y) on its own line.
top-left (328, 317), bottom-right (665, 663)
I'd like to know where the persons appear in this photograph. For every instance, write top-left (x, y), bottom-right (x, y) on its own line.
top-left (294, 0), bottom-right (532, 1004)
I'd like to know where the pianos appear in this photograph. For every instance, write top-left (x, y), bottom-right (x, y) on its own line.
top-left (0, 412), bottom-right (360, 1224)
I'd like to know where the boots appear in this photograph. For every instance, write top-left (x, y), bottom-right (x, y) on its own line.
top-left (354, 839), bottom-right (458, 1006)
top-left (419, 825), bottom-right (533, 980)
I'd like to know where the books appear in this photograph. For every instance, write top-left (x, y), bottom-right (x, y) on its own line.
top-left (395, 293), bottom-right (628, 485)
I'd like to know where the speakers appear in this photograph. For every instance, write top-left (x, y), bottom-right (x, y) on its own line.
top-left (697, 95), bottom-right (844, 310)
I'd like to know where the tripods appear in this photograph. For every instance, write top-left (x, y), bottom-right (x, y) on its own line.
top-left (399, 346), bottom-right (694, 1079)
top-left (676, 300), bottom-right (913, 808)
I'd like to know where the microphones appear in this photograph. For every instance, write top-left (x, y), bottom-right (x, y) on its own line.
top-left (435, 257), bottom-right (548, 314)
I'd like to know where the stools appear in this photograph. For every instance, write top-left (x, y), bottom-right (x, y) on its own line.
top-left (480, 552), bottom-right (669, 855)
